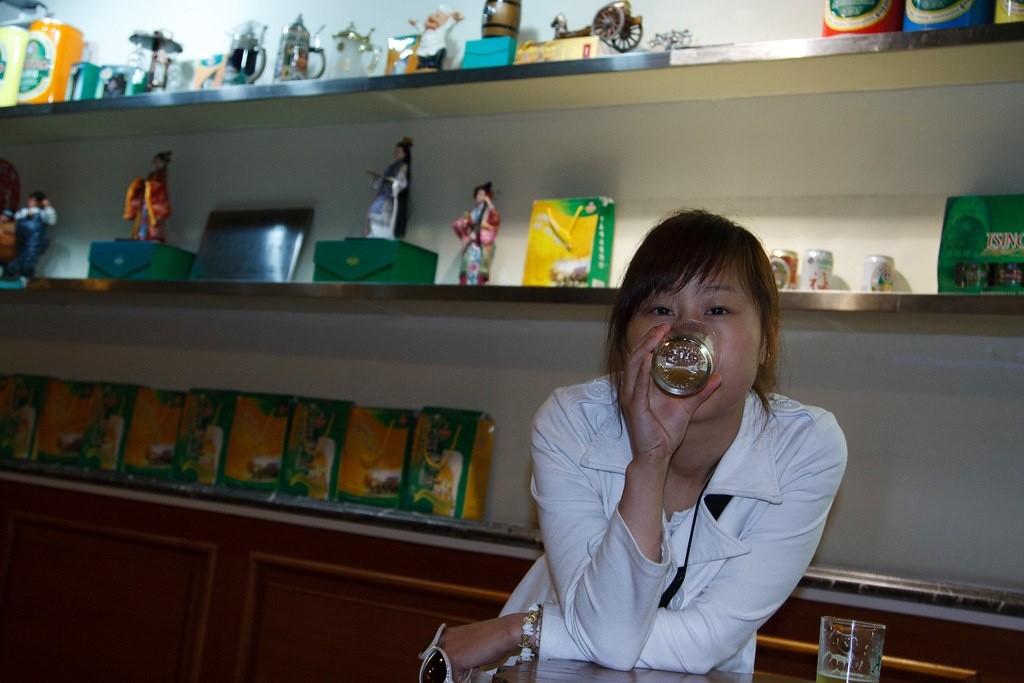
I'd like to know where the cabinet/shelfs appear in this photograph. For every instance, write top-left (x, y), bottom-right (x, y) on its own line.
top-left (0, 20), bottom-right (1024, 317)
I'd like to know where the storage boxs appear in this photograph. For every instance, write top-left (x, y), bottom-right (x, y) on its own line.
top-left (88, 240), bottom-right (196, 282)
top-left (936, 193), bottom-right (1024, 295)
top-left (314, 235), bottom-right (438, 286)
top-left (460, 36), bottom-right (518, 71)
top-left (0, 374), bottom-right (499, 524)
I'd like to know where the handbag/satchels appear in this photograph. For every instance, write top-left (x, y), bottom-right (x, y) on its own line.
top-left (521, 196), bottom-right (616, 287)
top-left (0, 373), bottom-right (496, 522)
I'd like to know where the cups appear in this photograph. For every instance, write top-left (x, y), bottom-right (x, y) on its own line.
top-left (816, 615), bottom-right (886, 683)
top-left (651, 319), bottom-right (719, 395)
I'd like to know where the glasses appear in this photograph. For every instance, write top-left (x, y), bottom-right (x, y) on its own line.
top-left (418, 623), bottom-right (474, 683)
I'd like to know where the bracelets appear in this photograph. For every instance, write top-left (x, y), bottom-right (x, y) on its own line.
top-left (519, 602), bottom-right (544, 664)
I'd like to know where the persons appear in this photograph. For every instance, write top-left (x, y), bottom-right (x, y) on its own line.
top-left (124, 147), bottom-right (174, 245)
top-left (452, 181), bottom-right (501, 285)
top-left (406, 5), bottom-right (464, 71)
top-left (421, 207), bottom-right (851, 683)
top-left (366, 136), bottom-right (414, 240)
top-left (0, 190), bottom-right (58, 289)
